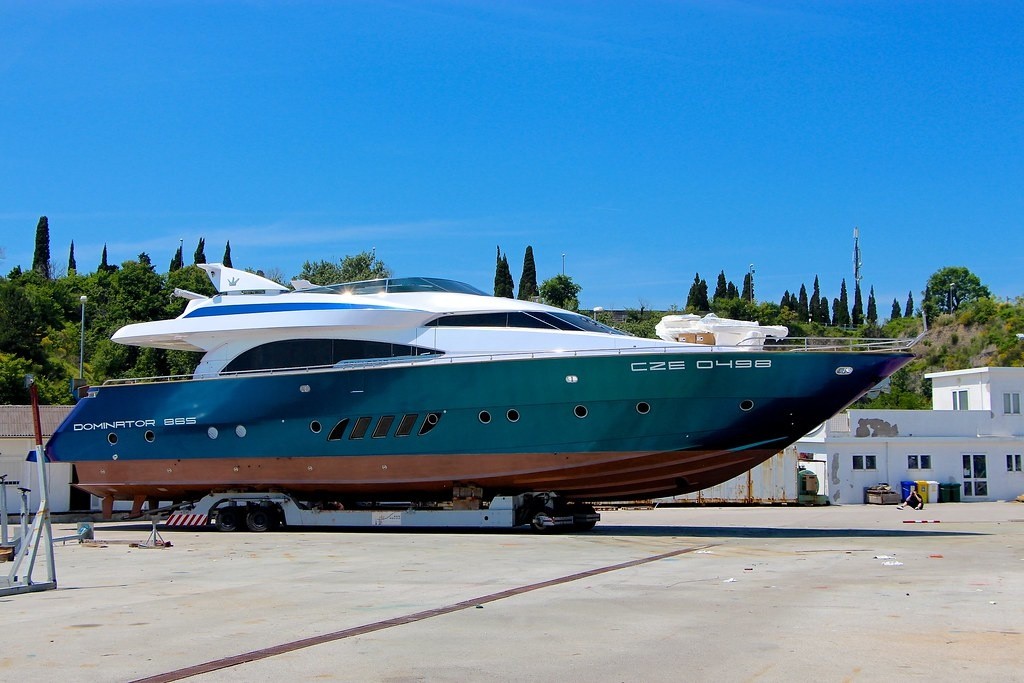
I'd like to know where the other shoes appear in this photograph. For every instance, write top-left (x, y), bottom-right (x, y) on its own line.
top-left (915, 507), bottom-right (921, 510)
top-left (897, 506), bottom-right (904, 510)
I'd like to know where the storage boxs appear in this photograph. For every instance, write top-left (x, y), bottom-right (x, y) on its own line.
top-left (678, 333), bottom-right (715, 346)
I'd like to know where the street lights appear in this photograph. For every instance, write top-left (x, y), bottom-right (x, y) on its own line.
top-left (79, 295), bottom-right (88, 380)
top-left (949, 283), bottom-right (956, 313)
top-left (749, 264), bottom-right (754, 304)
top-left (562, 253), bottom-right (566, 277)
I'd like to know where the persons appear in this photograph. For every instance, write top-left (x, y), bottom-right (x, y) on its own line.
top-left (896, 489), bottom-right (924, 510)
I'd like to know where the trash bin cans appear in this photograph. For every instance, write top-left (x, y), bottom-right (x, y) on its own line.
top-left (900, 480), bottom-right (916, 503)
top-left (951, 483), bottom-right (961, 502)
top-left (927, 481), bottom-right (940, 503)
top-left (914, 481), bottom-right (928, 503)
top-left (939, 482), bottom-right (951, 503)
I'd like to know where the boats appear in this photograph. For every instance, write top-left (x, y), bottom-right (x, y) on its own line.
top-left (44, 261), bottom-right (927, 502)
